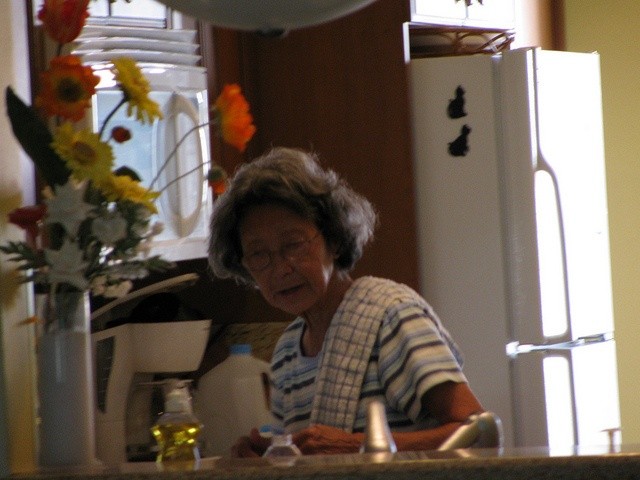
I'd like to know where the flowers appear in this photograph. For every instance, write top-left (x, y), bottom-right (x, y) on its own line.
top-left (0, 0), bottom-right (256, 328)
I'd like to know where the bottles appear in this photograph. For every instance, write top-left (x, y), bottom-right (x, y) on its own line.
top-left (152, 389), bottom-right (204, 461)
top-left (358, 385), bottom-right (398, 454)
top-left (259, 423), bottom-right (306, 457)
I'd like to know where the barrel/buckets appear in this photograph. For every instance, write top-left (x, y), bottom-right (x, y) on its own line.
top-left (196, 344), bottom-right (273, 456)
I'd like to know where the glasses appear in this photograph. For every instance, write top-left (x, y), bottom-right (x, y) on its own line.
top-left (238, 231), bottom-right (320, 273)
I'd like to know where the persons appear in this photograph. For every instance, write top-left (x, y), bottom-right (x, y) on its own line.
top-left (207, 147), bottom-right (484, 457)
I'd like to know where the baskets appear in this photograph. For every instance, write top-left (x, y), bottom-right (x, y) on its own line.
top-left (408, 23), bottom-right (516, 59)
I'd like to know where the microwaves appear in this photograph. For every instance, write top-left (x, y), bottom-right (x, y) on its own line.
top-left (40, 68), bottom-right (216, 275)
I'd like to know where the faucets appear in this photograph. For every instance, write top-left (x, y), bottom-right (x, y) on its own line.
top-left (358, 402), bottom-right (397, 452)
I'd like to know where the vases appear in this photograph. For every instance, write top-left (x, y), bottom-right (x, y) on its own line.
top-left (31, 290), bottom-right (99, 474)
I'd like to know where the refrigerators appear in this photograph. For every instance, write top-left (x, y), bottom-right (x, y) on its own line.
top-left (410, 46), bottom-right (622, 444)
